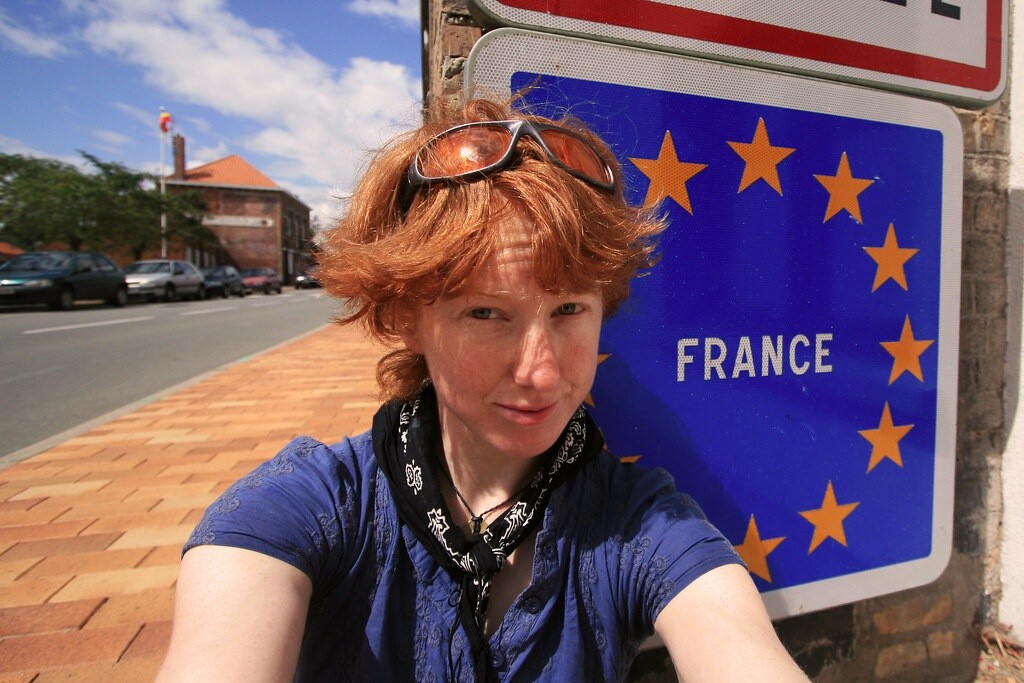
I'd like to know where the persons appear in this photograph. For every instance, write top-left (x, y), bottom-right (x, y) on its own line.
top-left (157, 103), bottom-right (810, 683)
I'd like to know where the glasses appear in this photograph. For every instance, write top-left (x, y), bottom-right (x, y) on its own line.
top-left (395, 119), bottom-right (617, 223)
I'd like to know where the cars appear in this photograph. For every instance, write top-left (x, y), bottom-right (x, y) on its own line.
top-left (295, 266), bottom-right (321, 289)
top-left (0, 250), bottom-right (128, 310)
top-left (239, 267), bottom-right (282, 294)
top-left (200, 265), bottom-right (247, 298)
top-left (125, 259), bottom-right (207, 302)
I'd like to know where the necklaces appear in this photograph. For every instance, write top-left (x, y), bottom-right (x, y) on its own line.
top-left (439, 465), bottom-right (532, 532)
